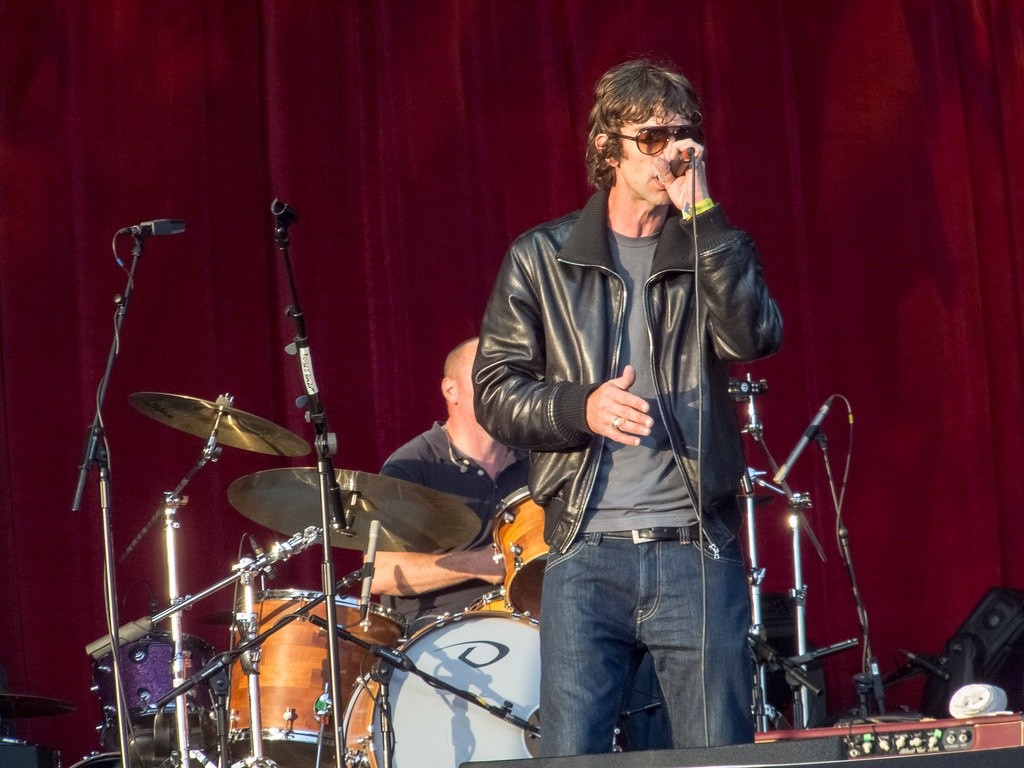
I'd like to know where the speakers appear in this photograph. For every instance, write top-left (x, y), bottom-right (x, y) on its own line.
top-left (918, 584), bottom-right (1024, 716)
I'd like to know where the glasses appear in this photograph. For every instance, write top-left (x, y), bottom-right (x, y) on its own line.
top-left (607, 125), bottom-right (703, 155)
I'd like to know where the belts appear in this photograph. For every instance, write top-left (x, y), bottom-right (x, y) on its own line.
top-left (587, 523), bottom-right (708, 542)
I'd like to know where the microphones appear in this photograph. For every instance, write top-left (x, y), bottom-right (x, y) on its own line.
top-left (119, 219), bottom-right (185, 235)
top-left (670, 148), bottom-right (696, 178)
top-left (773, 397), bottom-right (832, 484)
top-left (900, 651), bottom-right (951, 681)
top-left (360, 520), bottom-right (380, 619)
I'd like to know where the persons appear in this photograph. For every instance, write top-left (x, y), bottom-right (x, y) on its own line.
top-left (379, 337), bottom-right (529, 640)
top-left (471, 59), bottom-right (783, 757)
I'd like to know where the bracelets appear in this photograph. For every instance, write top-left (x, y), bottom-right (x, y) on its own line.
top-left (681, 197), bottom-right (714, 220)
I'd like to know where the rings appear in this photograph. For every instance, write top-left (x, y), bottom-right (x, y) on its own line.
top-left (612, 415), bottom-right (622, 429)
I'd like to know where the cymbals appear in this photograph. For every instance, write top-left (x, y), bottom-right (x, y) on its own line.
top-left (1, 692), bottom-right (78, 721)
top-left (736, 492), bottom-right (773, 515)
top-left (225, 466), bottom-right (482, 555)
top-left (127, 389), bottom-right (313, 457)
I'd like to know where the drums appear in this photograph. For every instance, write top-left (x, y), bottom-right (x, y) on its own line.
top-left (491, 484), bottom-right (550, 622)
top-left (92, 628), bottom-right (218, 752)
top-left (224, 588), bottom-right (408, 767)
top-left (342, 608), bottom-right (543, 768)
top-left (466, 585), bottom-right (515, 614)
